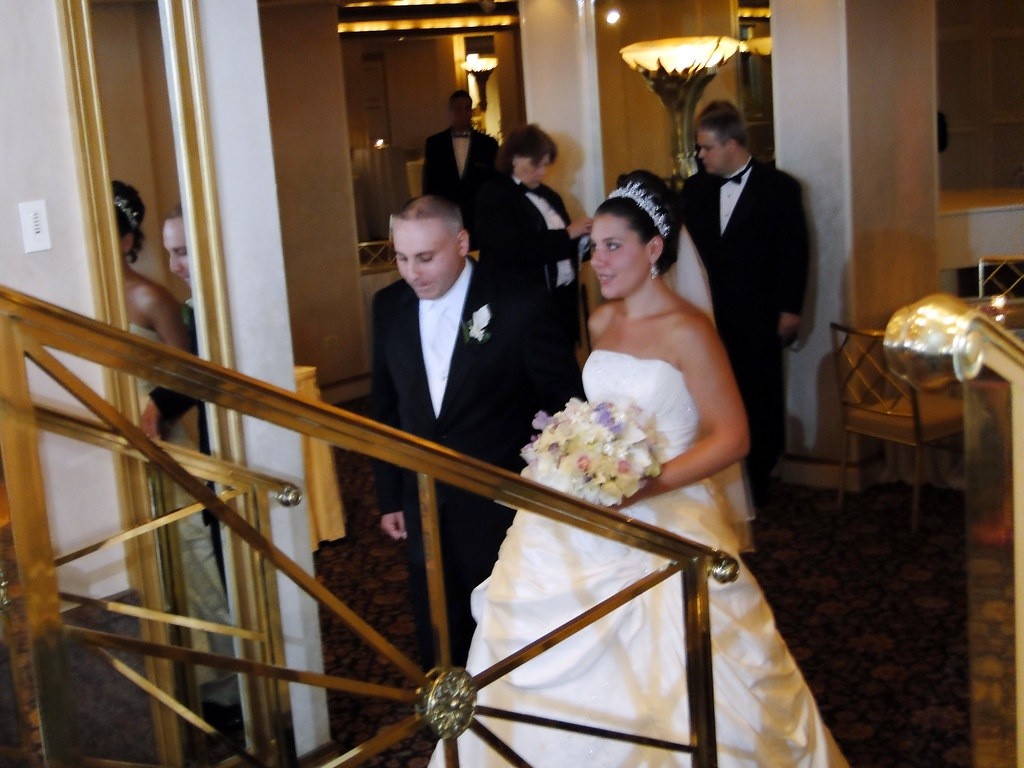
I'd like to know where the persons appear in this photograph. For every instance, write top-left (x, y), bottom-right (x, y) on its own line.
top-left (111, 179), bottom-right (241, 711)
top-left (368, 88), bottom-right (851, 768)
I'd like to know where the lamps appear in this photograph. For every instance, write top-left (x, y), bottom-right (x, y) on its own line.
top-left (738, 39), bottom-right (753, 89)
top-left (619, 34), bottom-right (739, 192)
top-left (461, 58), bottom-right (499, 112)
top-left (745, 37), bottom-right (772, 66)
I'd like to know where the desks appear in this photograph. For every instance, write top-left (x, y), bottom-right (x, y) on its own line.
top-left (961, 297), bottom-right (1024, 342)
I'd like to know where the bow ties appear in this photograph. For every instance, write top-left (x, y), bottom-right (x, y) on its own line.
top-left (519, 182), bottom-right (543, 198)
top-left (719, 157), bottom-right (753, 188)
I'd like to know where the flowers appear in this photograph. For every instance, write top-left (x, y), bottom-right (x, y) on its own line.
top-left (522, 397), bottom-right (662, 508)
top-left (459, 304), bottom-right (492, 346)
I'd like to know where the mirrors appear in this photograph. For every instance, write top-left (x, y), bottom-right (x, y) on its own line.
top-left (54, 1), bottom-right (282, 768)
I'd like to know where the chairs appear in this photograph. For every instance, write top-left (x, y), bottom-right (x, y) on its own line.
top-left (831, 323), bottom-right (964, 531)
top-left (979, 255), bottom-right (1024, 298)
